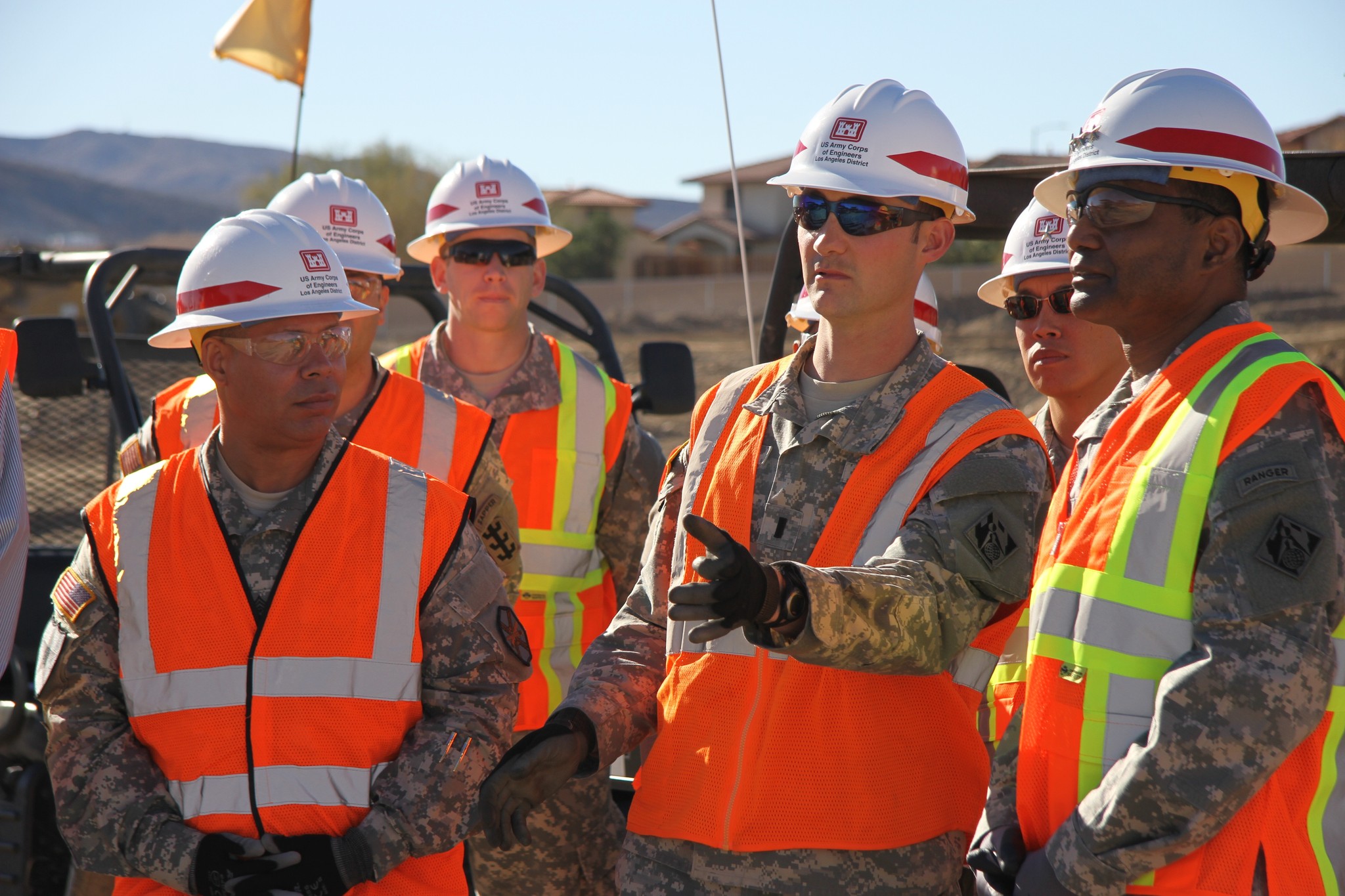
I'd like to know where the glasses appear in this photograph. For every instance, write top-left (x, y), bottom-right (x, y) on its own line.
top-left (1066, 181), bottom-right (1221, 229)
top-left (210, 326), bottom-right (353, 364)
top-left (346, 273), bottom-right (381, 302)
top-left (1004, 287), bottom-right (1075, 321)
top-left (441, 239), bottom-right (537, 267)
top-left (792, 188), bottom-right (938, 237)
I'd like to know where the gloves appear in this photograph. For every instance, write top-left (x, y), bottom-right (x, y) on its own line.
top-left (668, 513), bottom-right (779, 644)
top-left (477, 710), bottom-right (594, 851)
top-left (966, 824), bottom-right (1079, 896)
top-left (190, 828), bottom-right (363, 896)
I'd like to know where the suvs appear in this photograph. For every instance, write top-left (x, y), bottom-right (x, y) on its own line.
top-left (0, 240), bottom-right (694, 896)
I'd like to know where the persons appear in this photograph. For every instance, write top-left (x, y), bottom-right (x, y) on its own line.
top-left (777, 267), bottom-right (1014, 415)
top-left (0, 326), bottom-right (31, 687)
top-left (113, 165), bottom-right (525, 673)
top-left (20, 207), bottom-right (534, 896)
top-left (955, 64), bottom-right (1344, 896)
top-left (977, 165), bottom-right (1144, 759)
top-left (377, 150), bottom-right (682, 896)
top-left (472, 78), bottom-right (1058, 896)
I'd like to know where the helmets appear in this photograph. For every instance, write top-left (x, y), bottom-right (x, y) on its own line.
top-left (766, 78), bottom-right (976, 226)
top-left (265, 169), bottom-right (404, 281)
top-left (977, 195), bottom-right (1071, 309)
top-left (405, 153), bottom-right (572, 264)
top-left (1033, 67), bottom-right (1328, 248)
top-left (147, 208), bottom-right (380, 347)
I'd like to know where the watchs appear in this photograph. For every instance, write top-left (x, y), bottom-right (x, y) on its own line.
top-left (764, 562), bottom-right (807, 631)
